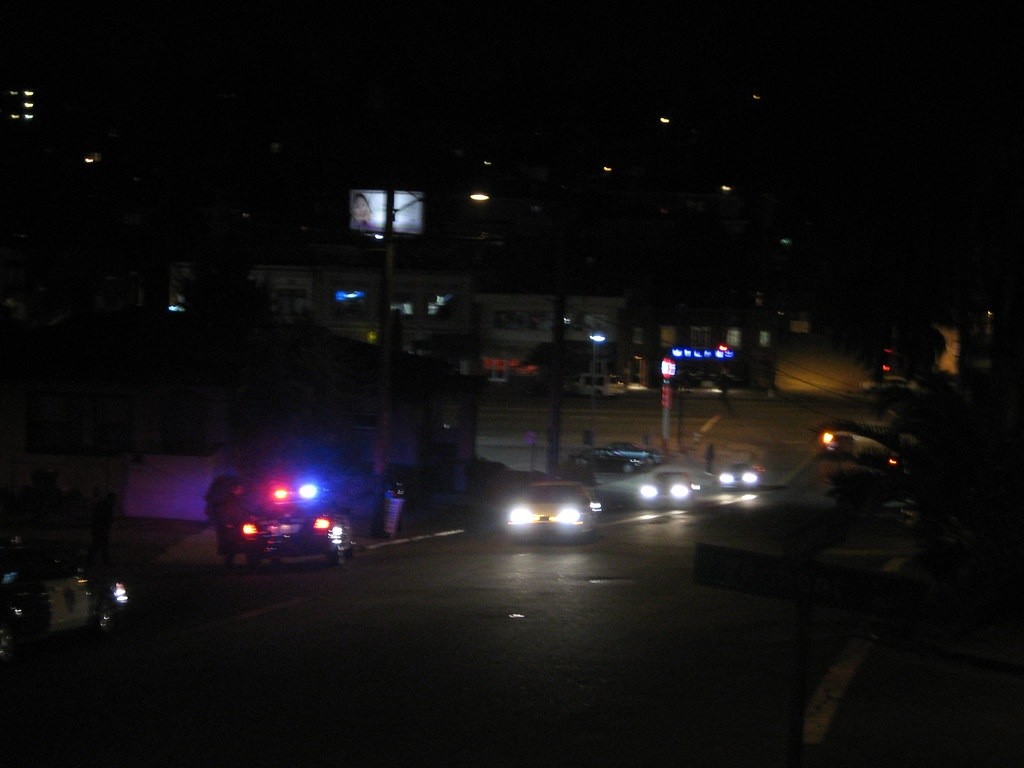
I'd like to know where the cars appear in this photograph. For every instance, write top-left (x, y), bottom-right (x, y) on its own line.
top-left (639, 463), bottom-right (701, 505)
top-left (0, 538), bottom-right (128, 671)
top-left (564, 372), bottom-right (625, 400)
top-left (233, 476), bottom-right (354, 569)
top-left (720, 463), bottom-right (765, 492)
top-left (577, 440), bottom-right (664, 474)
top-left (509, 479), bottom-right (600, 544)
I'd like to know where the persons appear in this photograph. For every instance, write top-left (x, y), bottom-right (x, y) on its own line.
top-left (349, 194), bottom-right (379, 232)
